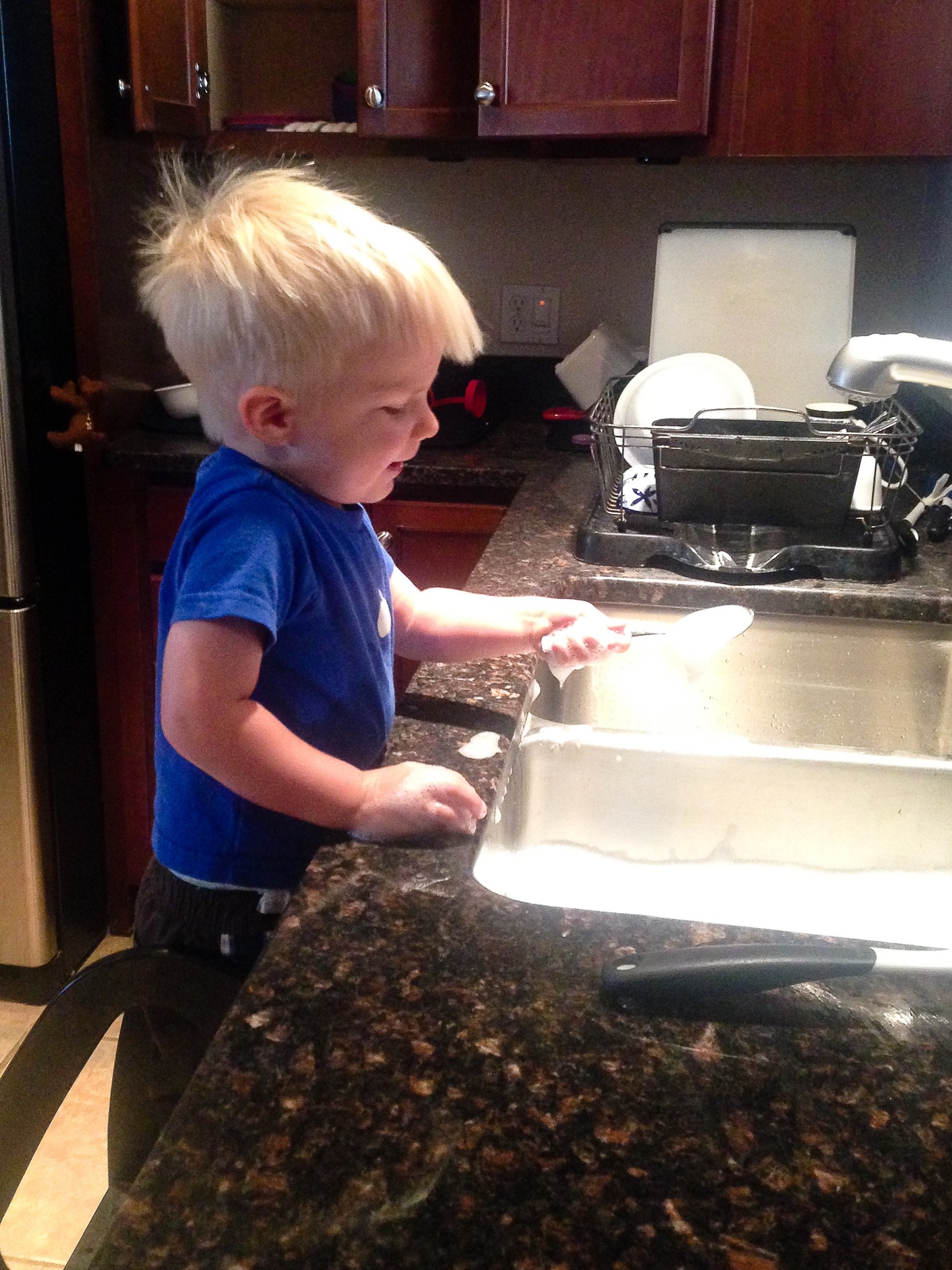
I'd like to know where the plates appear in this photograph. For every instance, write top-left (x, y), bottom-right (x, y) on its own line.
top-left (613, 353), bottom-right (758, 467)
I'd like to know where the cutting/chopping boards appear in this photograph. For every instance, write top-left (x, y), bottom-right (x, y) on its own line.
top-left (649, 223), bottom-right (856, 423)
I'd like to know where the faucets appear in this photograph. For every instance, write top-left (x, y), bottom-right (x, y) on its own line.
top-left (827, 331), bottom-right (952, 396)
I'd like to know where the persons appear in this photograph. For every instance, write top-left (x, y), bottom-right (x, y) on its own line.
top-left (108, 160), bottom-right (628, 1188)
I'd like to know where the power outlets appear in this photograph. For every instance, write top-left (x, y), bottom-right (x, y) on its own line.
top-left (500, 284), bottom-right (561, 344)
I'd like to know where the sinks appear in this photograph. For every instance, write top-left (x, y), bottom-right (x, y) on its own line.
top-left (464, 715), bottom-right (949, 953)
top-left (524, 569), bottom-right (952, 785)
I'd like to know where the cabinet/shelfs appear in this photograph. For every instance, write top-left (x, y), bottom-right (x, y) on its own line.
top-left (116, 0), bottom-right (952, 158)
top-left (91, 487), bottom-right (508, 936)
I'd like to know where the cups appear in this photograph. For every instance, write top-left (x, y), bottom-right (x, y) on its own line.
top-left (850, 452), bottom-right (884, 511)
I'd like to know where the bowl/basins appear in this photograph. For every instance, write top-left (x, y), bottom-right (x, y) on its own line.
top-left (617, 464), bottom-right (657, 514)
top-left (154, 384), bottom-right (198, 418)
top-left (805, 402), bottom-right (858, 418)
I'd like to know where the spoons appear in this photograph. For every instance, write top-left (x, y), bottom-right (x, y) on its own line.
top-left (630, 604), bottom-right (753, 642)
